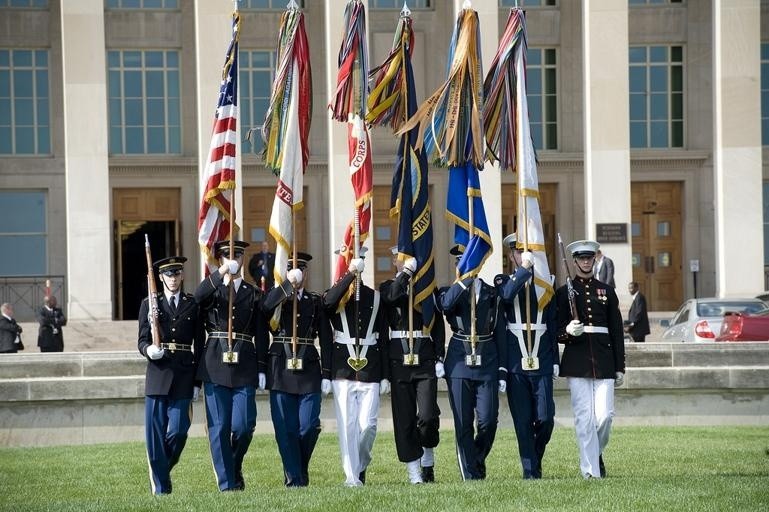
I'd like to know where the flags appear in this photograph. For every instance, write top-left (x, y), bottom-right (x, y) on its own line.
top-left (365, 12), bottom-right (440, 338)
top-left (198, 11), bottom-right (241, 280)
top-left (257, 3), bottom-right (316, 334)
top-left (328, 0), bottom-right (372, 316)
top-left (481, 9), bottom-right (556, 313)
top-left (395, 10), bottom-right (494, 288)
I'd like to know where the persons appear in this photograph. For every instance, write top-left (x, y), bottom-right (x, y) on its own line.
top-left (36, 295), bottom-right (66, 353)
top-left (0, 302), bottom-right (24, 354)
top-left (264, 252), bottom-right (333, 490)
top-left (138, 254), bottom-right (207, 496)
top-left (379, 247), bottom-right (447, 486)
top-left (592, 249), bottom-right (616, 291)
top-left (494, 234), bottom-right (560, 480)
top-left (624, 280), bottom-right (653, 343)
top-left (439, 244), bottom-right (508, 483)
top-left (324, 248), bottom-right (392, 486)
top-left (247, 241), bottom-right (276, 292)
top-left (193, 239), bottom-right (270, 494)
top-left (555, 239), bottom-right (626, 482)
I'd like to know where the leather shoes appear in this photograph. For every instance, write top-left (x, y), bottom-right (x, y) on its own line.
top-left (598, 453), bottom-right (606, 478)
top-left (420, 466), bottom-right (434, 483)
top-left (359, 470), bottom-right (365, 484)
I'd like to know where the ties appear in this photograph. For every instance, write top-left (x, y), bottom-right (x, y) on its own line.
top-left (229, 281), bottom-right (236, 297)
top-left (594, 262), bottom-right (597, 278)
top-left (170, 297), bottom-right (176, 313)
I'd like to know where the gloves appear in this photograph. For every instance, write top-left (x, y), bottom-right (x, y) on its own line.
top-left (146, 344), bottom-right (165, 361)
top-left (286, 268), bottom-right (302, 284)
top-left (566, 319), bottom-right (585, 337)
top-left (349, 257), bottom-right (365, 273)
top-left (226, 260), bottom-right (238, 274)
top-left (403, 257), bottom-right (417, 277)
top-left (519, 250), bottom-right (536, 270)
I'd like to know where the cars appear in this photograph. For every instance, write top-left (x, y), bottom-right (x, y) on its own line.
top-left (716, 305), bottom-right (769, 343)
top-left (661, 298), bottom-right (767, 343)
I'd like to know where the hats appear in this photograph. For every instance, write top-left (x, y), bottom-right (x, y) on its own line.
top-left (285, 251), bottom-right (313, 267)
top-left (449, 244), bottom-right (465, 258)
top-left (153, 257), bottom-right (187, 276)
top-left (566, 240), bottom-right (600, 259)
top-left (503, 232), bottom-right (516, 248)
top-left (334, 246), bottom-right (368, 258)
top-left (213, 240), bottom-right (250, 258)
top-left (388, 245), bottom-right (398, 255)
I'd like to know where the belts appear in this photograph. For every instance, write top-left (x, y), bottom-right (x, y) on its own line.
top-left (273, 336), bottom-right (315, 344)
top-left (157, 342), bottom-right (193, 352)
top-left (583, 327), bottom-right (608, 333)
top-left (506, 323), bottom-right (547, 331)
top-left (208, 331), bottom-right (253, 342)
top-left (391, 330), bottom-right (431, 338)
top-left (334, 337), bottom-right (378, 345)
top-left (452, 332), bottom-right (494, 343)
